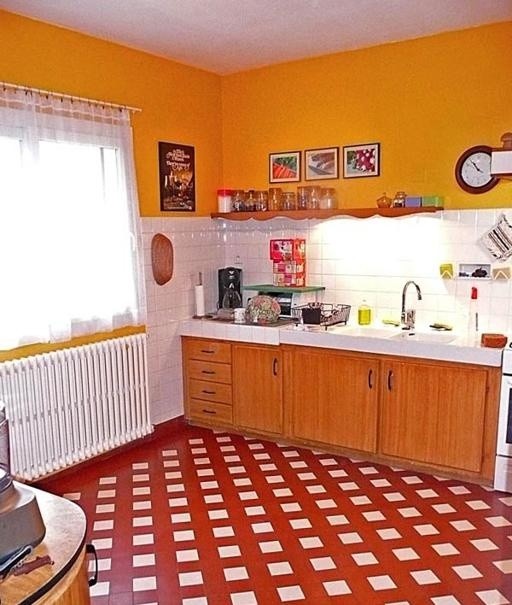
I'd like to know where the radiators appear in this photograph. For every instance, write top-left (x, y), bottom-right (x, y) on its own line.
top-left (0, 333), bottom-right (155, 483)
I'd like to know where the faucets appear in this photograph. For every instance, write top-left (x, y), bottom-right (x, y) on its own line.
top-left (401, 281), bottom-right (422, 329)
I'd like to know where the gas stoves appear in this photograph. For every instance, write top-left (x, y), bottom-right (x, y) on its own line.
top-left (502, 338), bottom-right (512, 375)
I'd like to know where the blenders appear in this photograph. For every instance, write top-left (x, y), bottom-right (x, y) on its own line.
top-left (0, 402), bottom-right (44, 556)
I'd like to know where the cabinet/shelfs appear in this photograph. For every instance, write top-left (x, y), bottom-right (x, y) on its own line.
top-left (230, 342), bottom-right (290, 443)
top-left (281, 345), bottom-right (498, 489)
top-left (0, 478), bottom-right (99, 605)
top-left (179, 336), bottom-right (235, 433)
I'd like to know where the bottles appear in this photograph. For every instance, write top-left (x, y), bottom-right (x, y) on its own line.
top-left (466, 287), bottom-right (479, 340)
top-left (377, 192), bottom-right (393, 208)
top-left (358, 299), bottom-right (371, 325)
top-left (217, 186), bottom-right (338, 213)
top-left (394, 190), bottom-right (407, 207)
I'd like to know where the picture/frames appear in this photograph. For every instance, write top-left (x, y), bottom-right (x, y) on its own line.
top-left (342, 142), bottom-right (380, 178)
top-left (157, 140), bottom-right (198, 212)
top-left (268, 150), bottom-right (300, 183)
top-left (304, 146), bottom-right (339, 180)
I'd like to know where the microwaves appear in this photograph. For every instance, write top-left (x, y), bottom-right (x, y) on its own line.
top-left (256, 289), bottom-right (316, 319)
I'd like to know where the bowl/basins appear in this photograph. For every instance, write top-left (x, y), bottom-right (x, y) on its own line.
top-left (481, 334), bottom-right (508, 348)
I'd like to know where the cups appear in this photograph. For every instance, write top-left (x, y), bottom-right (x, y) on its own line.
top-left (440, 263), bottom-right (453, 278)
top-left (232, 308), bottom-right (246, 323)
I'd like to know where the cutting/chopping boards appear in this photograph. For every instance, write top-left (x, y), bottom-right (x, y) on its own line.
top-left (233, 318), bottom-right (295, 326)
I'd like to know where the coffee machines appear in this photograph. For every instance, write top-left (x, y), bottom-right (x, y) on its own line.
top-left (217, 266), bottom-right (244, 310)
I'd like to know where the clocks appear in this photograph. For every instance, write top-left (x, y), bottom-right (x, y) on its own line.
top-left (454, 145), bottom-right (502, 192)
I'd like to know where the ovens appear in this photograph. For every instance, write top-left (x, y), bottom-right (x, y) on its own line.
top-left (494, 376), bottom-right (512, 494)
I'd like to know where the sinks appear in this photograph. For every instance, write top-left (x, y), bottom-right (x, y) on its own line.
top-left (388, 331), bottom-right (459, 345)
